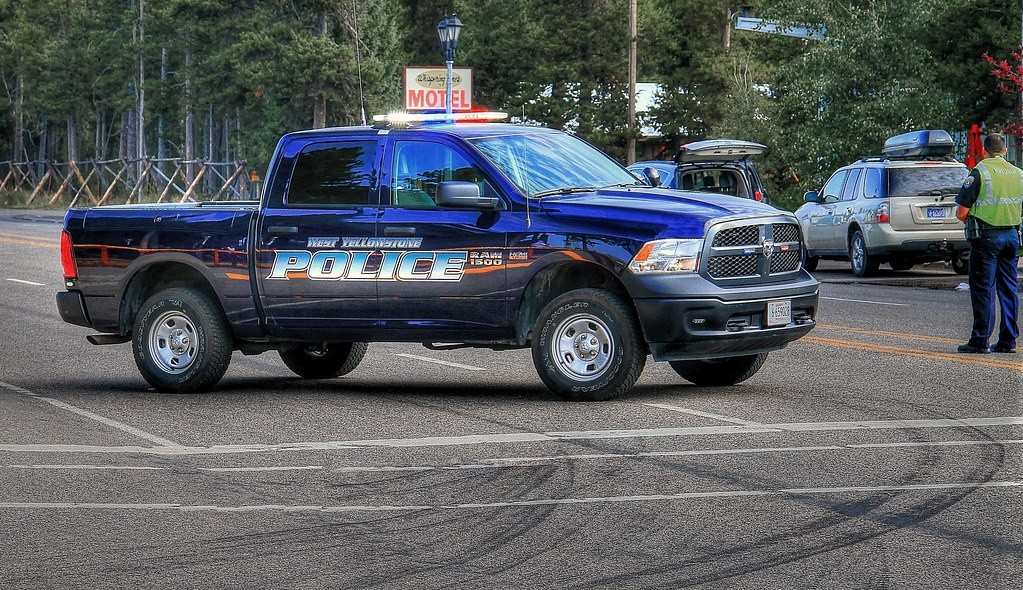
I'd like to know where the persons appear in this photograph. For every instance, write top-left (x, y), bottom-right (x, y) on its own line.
top-left (953, 132), bottom-right (1023, 353)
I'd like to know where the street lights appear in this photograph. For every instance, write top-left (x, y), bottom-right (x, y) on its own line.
top-left (437, 10), bottom-right (467, 184)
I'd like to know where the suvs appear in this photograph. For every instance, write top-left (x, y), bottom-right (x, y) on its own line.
top-left (792, 156), bottom-right (973, 276)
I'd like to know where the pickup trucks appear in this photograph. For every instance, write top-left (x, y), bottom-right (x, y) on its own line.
top-left (55, 110), bottom-right (821, 399)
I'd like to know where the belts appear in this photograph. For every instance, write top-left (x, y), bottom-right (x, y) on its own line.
top-left (981, 224), bottom-right (1015, 229)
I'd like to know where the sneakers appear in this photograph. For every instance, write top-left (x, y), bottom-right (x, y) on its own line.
top-left (958, 342), bottom-right (1017, 354)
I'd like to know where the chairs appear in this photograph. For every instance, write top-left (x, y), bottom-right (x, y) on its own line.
top-left (398, 155), bottom-right (436, 206)
top-left (699, 176), bottom-right (720, 192)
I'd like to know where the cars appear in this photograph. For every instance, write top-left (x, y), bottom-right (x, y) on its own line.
top-left (626, 138), bottom-right (768, 203)
